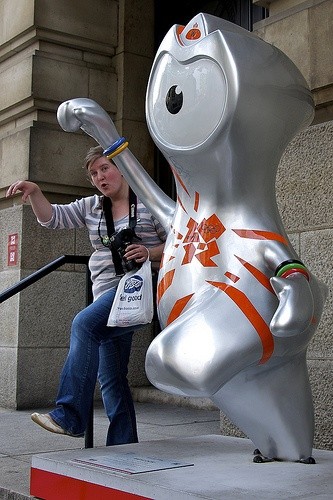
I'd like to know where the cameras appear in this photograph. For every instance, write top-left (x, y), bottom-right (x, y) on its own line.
top-left (109, 229), bottom-right (135, 271)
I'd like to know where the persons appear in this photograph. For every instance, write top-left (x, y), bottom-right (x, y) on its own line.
top-left (5, 144), bottom-right (169, 447)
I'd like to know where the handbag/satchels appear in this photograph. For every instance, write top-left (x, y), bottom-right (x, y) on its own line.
top-left (106, 246), bottom-right (154, 327)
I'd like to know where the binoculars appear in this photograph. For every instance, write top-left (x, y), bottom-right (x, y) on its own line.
top-left (108, 229), bottom-right (137, 277)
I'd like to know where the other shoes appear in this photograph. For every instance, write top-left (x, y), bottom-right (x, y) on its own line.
top-left (31, 412), bottom-right (73, 436)
